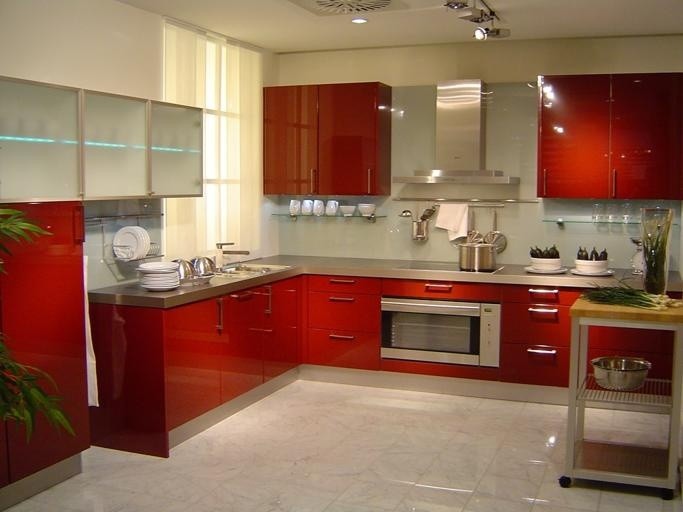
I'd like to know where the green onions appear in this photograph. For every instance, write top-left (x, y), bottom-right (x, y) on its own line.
top-left (579, 275), bottom-right (683, 311)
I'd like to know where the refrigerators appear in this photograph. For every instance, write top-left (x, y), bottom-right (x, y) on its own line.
top-left (0, 198), bottom-right (91, 511)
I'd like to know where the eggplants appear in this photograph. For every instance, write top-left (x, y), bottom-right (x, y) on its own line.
top-left (578, 246), bottom-right (607, 261)
top-left (529, 244), bottom-right (559, 258)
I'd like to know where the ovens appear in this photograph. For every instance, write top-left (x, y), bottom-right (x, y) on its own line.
top-left (376, 296), bottom-right (502, 369)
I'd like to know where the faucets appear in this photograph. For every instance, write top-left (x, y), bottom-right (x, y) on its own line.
top-left (215, 242), bottom-right (250, 268)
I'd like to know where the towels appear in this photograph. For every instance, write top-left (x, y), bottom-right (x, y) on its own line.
top-left (433, 205), bottom-right (470, 243)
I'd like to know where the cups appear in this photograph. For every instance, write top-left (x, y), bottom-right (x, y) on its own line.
top-left (591, 202), bottom-right (633, 223)
top-left (288, 200), bottom-right (338, 216)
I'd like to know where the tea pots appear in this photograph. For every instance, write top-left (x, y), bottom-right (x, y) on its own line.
top-left (629, 237), bottom-right (644, 275)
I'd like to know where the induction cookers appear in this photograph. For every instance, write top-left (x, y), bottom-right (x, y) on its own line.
top-left (390, 260), bottom-right (504, 276)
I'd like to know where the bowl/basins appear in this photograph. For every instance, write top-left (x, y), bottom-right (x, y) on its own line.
top-left (574, 261), bottom-right (611, 273)
top-left (339, 205), bottom-right (355, 217)
top-left (588, 354), bottom-right (652, 389)
top-left (358, 204), bottom-right (377, 217)
top-left (530, 256), bottom-right (561, 270)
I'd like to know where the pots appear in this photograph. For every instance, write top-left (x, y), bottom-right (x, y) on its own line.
top-left (459, 243), bottom-right (498, 271)
top-left (461, 207), bottom-right (507, 256)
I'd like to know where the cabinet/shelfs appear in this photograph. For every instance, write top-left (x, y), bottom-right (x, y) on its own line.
top-left (87, 296), bottom-right (222, 457)
top-left (0, 75), bottom-right (82, 205)
top-left (149, 101), bottom-right (202, 198)
top-left (80, 88), bottom-right (150, 201)
top-left (611, 73), bottom-right (682, 199)
top-left (500, 284), bottom-right (599, 389)
top-left (262, 84), bottom-right (317, 195)
top-left (558, 291), bottom-right (682, 499)
top-left (318, 82), bottom-right (390, 196)
top-left (381, 277), bottom-right (502, 303)
top-left (220, 286), bottom-right (263, 404)
top-left (536, 75), bottom-right (610, 198)
top-left (264, 275), bottom-right (296, 383)
top-left (307, 275), bottom-right (381, 369)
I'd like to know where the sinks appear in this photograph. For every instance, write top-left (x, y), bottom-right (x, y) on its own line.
top-left (222, 261), bottom-right (297, 272)
top-left (213, 268), bottom-right (273, 279)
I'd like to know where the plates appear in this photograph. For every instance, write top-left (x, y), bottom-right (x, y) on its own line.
top-left (524, 266), bottom-right (567, 274)
top-left (136, 254), bottom-right (214, 293)
top-left (111, 225), bottom-right (150, 263)
top-left (568, 269), bottom-right (614, 276)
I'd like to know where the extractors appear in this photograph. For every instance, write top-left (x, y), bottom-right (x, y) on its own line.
top-left (391, 78), bottom-right (520, 185)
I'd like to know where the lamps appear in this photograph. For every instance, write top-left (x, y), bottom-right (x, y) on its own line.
top-left (473, 22), bottom-right (488, 42)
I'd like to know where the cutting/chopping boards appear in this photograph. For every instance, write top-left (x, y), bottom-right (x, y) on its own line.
top-left (568, 292), bottom-right (682, 326)
